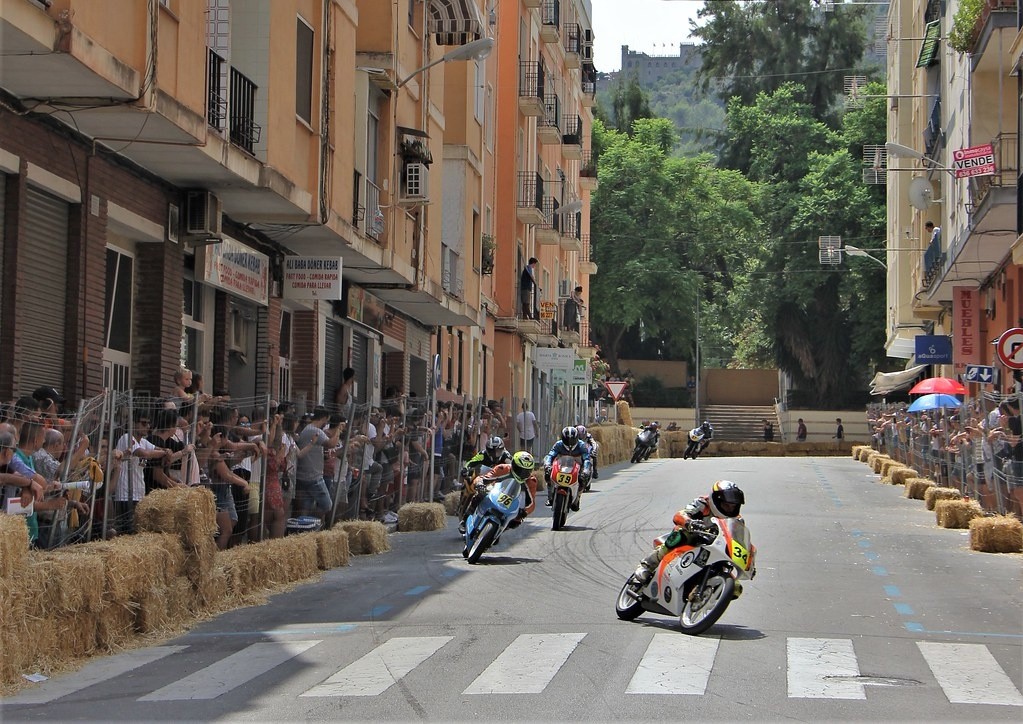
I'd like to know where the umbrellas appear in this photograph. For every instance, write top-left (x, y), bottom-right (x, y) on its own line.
top-left (906, 378), bottom-right (969, 413)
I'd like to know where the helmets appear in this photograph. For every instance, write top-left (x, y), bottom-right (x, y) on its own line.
top-left (709, 482), bottom-right (744, 518)
top-left (485, 437), bottom-right (505, 459)
top-left (650, 422), bottom-right (658, 431)
top-left (576, 425), bottom-right (587, 439)
top-left (511, 450), bottom-right (535, 484)
top-left (703, 422), bottom-right (710, 430)
top-left (561, 427), bottom-right (579, 446)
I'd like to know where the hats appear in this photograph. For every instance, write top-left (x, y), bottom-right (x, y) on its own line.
top-left (32, 386), bottom-right (67, 404)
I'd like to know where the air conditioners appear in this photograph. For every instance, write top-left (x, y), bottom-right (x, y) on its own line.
top-left (406, 164), bottom-right (429, 202)
top-left (186, 190), bottom-right (222, 240)
top-left (584, 47), bottom-right (593, 62)
top-left (585, 29), bottom-right (593, 45)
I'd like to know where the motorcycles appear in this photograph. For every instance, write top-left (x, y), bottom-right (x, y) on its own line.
top-left (631, 429), bottom-right (659, 464)
top-left (545, 455), bottom-right (586, 530)
top-left (459, 478), bottom-right (525, 565)
top-left (455, 463), bottom-right (494, 525)
top-left (683, 429), bottom-right (708, 460)
top-left (616, 517), bottom-right (757, 636)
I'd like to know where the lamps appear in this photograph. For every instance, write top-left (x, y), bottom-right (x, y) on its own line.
top-left (844, 245), bottom-right (887, 269)
top-left (398, 37), bottom-right (494, 94)
top-left (529, 200), bottom-right (583, 229)
top-left (885, 141), bottom-right (958, 179)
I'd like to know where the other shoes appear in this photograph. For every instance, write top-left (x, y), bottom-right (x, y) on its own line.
top-left (450, 479), bottom-right (464, 488)
top-left (374, 510), bottom-right (399, 522)
top-left (437, 491), bottom-right (447, 499)
top-left (360, 507), bottom-right (373, 516)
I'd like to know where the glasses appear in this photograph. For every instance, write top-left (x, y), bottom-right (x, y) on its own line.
top-left (137, 420), bottom-right (152, 427)
top-left (238, 421), bottom-right (252, 428)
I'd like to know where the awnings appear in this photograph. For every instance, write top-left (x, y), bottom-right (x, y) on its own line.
top-left (427, 0), bottom-right (486, 46)
top-left (869, 364), bottom-right (929, 395)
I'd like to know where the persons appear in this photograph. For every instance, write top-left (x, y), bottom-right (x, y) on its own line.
top-left (760, 419), bottom-right (773, 441)
top-left (796, 418), bottom-right (807, 442)
top-left (516, 402), bottom-right (539, 452)
top-left (924, 219), bottom-right (941, 244)
top-left (832, 418), bottom-right (845, 440)
top-left (521, 257), bottom-right (540, 320)
top-left (565, 286), bottom-right (587, 334)
top-left (690, 421), bottom-right (714, 453)
top-left (632, 422), bottom-right (660, 456)
top-left (458, 422), bottom-right (599, 546)
top-left (0, 368), bottom-right (509, 552)
top-left (635, 480), bottom-right (761, 587)
top-left (867, 392), bottom-right (1022, 522)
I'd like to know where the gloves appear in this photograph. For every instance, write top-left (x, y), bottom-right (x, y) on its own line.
top-left (460, 468), bottom-right (468, 476)
top-left (686, 519), bottom-right (704, 531)
top-left (545, 466), bottom-right (552, 474)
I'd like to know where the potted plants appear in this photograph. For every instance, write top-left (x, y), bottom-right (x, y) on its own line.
top-left (946, 0), bottom-right (1015, 54)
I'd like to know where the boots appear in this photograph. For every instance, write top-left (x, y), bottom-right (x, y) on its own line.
top-left (545, 486), bottom-right (553, 505)
top-left (458, 501), bottom-right (475, 533)
top-left (573, 489), bottom-right (585, 511)
top-left (636, 545), bottom-right (670, 582)
